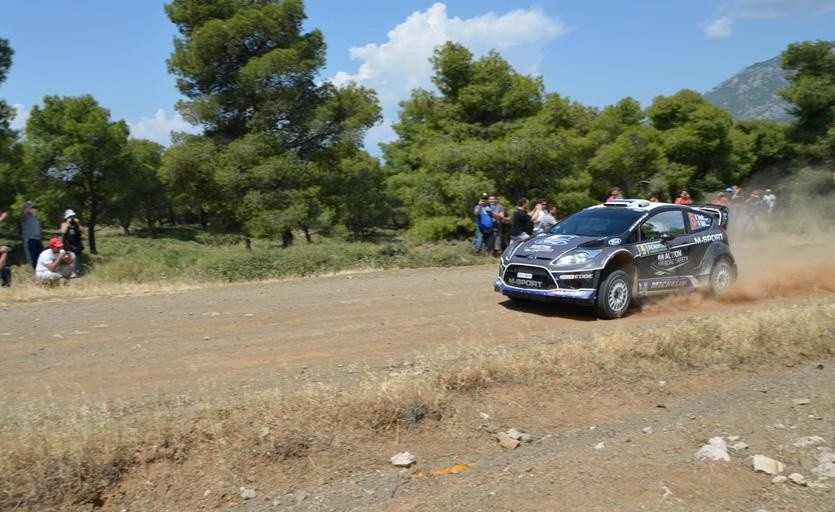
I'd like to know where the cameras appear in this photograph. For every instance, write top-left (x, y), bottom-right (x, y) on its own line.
top-left (64, 255), bottom-right (68, 260)
top-left (69, 217), bottom-right (76, 226)
top-left (31, 205), bottom-right (37, 209)
top-left (484, 201), bottom-right (488, 204)
top-left (7, 247), bottom-right (13, 255)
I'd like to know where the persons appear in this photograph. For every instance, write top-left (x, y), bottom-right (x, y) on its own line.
top-left (0, 245), bottom-right (12, 287)
top-left (19, 199), bottom-right (45, 270)
top-left (33, 237), bottom-right (72, 288)
top-left (59, 207), bottom-right (85, 279)
top-left (0, 208), bottom-right (7, 223)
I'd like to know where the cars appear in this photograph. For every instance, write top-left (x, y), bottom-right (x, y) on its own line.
top-left (493, 199), bottom-right (738, 321)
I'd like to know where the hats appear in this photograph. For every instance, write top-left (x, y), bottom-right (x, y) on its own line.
top-left (49, 238), bottom-right (64, 249)
top-left (24, 201), bottom-right (37, 208)
top-left (482, 192), bottom-right (488, 199)
top-left (725, 187), bottom-right (732, 192)
top-left (64, 209), bottom-right (75, 220)
